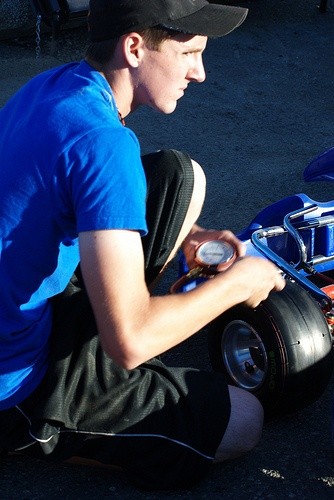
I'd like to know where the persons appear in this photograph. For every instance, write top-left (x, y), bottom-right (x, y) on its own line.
top-left (0, 0), bottom-right (288, 492)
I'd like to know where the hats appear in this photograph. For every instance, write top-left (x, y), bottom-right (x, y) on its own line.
top-left (88, 0), bottom-right (249, 39)
